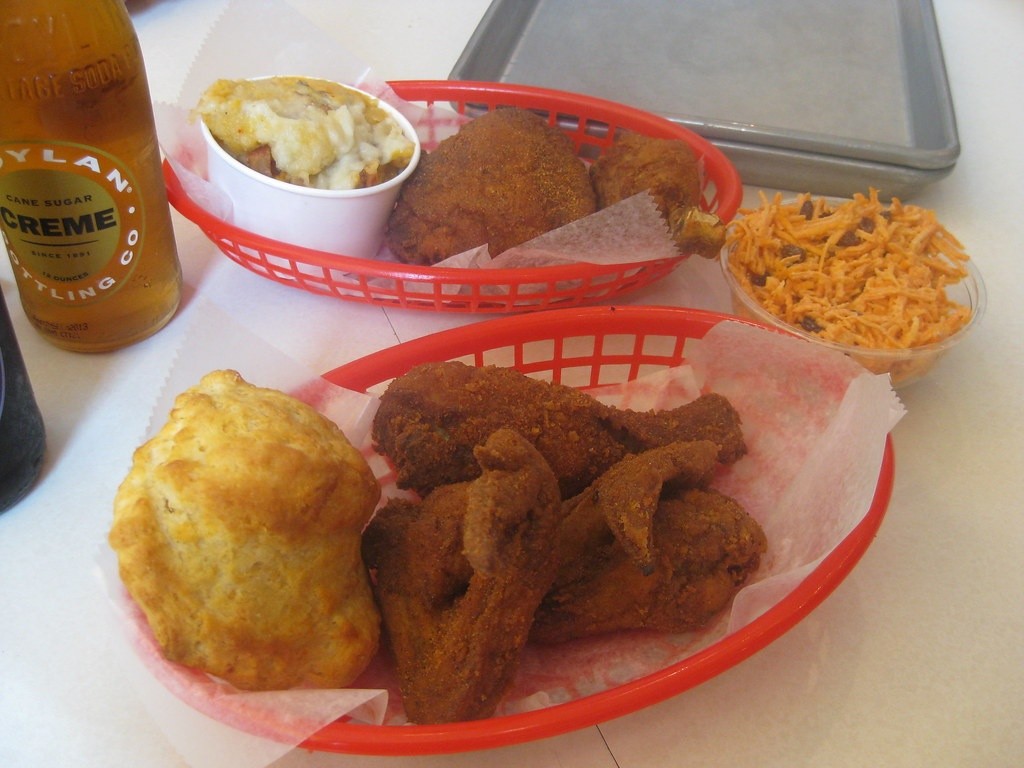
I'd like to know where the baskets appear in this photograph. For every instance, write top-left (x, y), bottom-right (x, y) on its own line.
top-left (122, 304), bottom-right (894, 756)
top-left (157, 80), bottom-right (745, 315)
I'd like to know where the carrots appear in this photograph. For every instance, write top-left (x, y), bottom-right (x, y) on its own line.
top-left (722, 189), bottom-right (972, 385)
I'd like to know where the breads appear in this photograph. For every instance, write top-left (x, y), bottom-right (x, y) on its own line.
top-left (109, 367), bottom-right (381, 691)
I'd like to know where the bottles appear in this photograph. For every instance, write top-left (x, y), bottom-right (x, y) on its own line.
top-left (0, 285), bottom-right (50, 515)
top-left (1, 0), bottom-right (183, 354)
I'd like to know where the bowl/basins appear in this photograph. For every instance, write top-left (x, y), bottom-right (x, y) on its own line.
top-left (199, 72), bottom-right (421, 282)
top-left (719, 193), bottom-right (987, 398)
